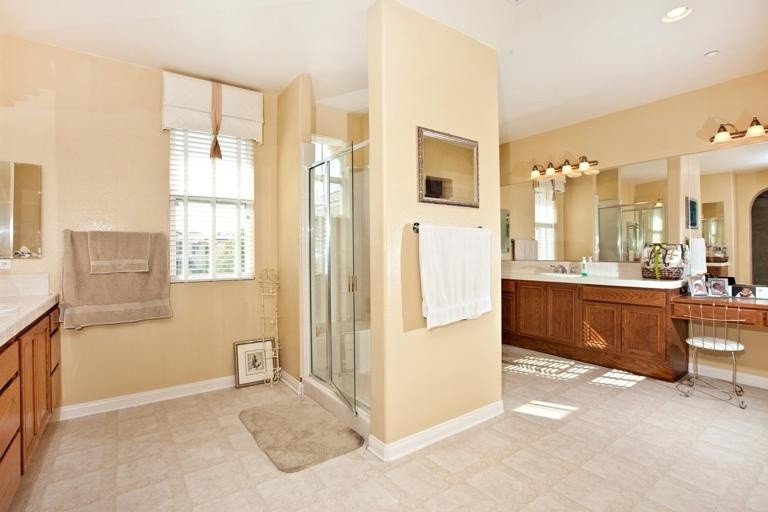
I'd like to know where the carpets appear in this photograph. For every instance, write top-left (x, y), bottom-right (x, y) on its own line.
top-left (240, 395), bottom-right (365, 473)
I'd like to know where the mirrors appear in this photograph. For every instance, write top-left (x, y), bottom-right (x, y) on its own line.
top-left (501, 143), bottom-right (768, 287)
top-left (418, 126), bottom-right (479, 207)
top-left (734, 170), bottom-right (768, 286)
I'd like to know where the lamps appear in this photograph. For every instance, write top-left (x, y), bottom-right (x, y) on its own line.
top-left (710, 117), bottom-right (768, 145)
top-left (529, 155), bottom-right (599, 182)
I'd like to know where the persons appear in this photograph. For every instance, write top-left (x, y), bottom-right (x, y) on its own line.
top-left (736, 288), bottom-right (755, 298)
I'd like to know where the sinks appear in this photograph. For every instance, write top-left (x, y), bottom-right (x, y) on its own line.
top-left (542, 273), bottom-right (582, 278)
top-left (1, 303), bottom-right (20, 315)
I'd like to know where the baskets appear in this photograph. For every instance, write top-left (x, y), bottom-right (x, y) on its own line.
top-left (641, 267), bottom-right (682, 279)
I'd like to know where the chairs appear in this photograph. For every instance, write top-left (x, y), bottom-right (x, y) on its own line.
top-left (678, 300), bottom-right (748, 410)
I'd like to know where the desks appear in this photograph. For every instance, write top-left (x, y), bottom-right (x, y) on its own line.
top-left (671, 288), bottom-right (768, 333)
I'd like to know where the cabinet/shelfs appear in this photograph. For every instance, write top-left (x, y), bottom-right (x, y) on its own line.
top-left (502, 279), bottom-right (517, 347)
top-left (49, 303), bottom-right (63, 415)
top-left (1, 336), bottom-right (22, 512)
top-left (579, 284), bottom-right (689, 383)
top-left (18, 315), bottom-right (51, 476)
top-left (516, 281), bottom-right (578, 361)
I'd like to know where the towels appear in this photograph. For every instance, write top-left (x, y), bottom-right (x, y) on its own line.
top-left (415, 227), bottom-right (493, 328)
top-left (57, 230), bottom-right (174, 330)
top-left (689, 237), bottom-right (708, 277)
top-left (511, 239), bottom-right (538, 261)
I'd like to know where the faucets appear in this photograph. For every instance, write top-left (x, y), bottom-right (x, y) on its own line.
top-left (550, 264), bottom-right (567, 273)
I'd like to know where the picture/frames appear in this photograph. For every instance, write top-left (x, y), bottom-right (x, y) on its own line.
top-left (686, 275), bottom-right (708, 297)
top-left (707, 278), bottom-right (728, 298)
top-left (687, 196), bottom-right (699, 229)
top-left (233, 338), bottom-right (278, 389)
